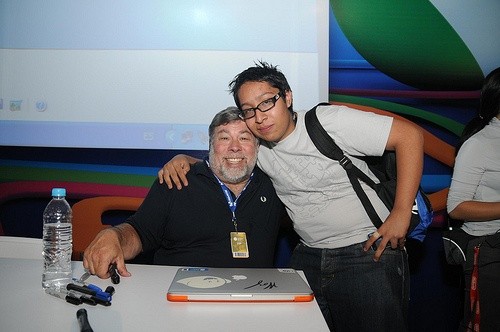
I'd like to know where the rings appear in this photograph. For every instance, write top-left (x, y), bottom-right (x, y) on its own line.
top-left (373, 231), bottom-right (381, 238)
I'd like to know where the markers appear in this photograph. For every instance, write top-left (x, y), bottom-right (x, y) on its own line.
top-left (76, 308), bottom-right (93, 332)
top-left (45, 283), bottom-right (111, 307)
top-left (109, 264), bottom-right (121, 285)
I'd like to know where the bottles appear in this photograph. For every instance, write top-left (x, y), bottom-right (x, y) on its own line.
top-left (41, 188), bottom-right (74, 289)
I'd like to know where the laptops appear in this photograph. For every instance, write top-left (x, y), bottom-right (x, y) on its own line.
top-left (167, 267), bottom-right (313, 301)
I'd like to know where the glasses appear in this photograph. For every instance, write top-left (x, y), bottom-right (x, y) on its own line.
top-left (237, 90), bottom-right (285, 122)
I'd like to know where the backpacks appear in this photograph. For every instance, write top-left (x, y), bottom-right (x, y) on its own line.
top-left (304, 102), bottom-right (434, 242)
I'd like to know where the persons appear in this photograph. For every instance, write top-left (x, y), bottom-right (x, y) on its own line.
top-left (445, 68), bottom-right (500, 332)
top-left (82, 107), bottom-right (285, 279)
top-left (225, 61), bottom-right (424, 332)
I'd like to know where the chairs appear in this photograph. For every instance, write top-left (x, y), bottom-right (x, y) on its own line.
top-left (69, 196), bottom-right (144, 261)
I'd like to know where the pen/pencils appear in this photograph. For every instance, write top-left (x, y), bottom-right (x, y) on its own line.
top-left (73, 277), bottom-right (101, 292)
top-left (80, 271), bottom-right (90, 280)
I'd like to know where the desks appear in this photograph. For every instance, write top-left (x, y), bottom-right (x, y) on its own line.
top-left (0, 257), bottom-right (332, 332)
